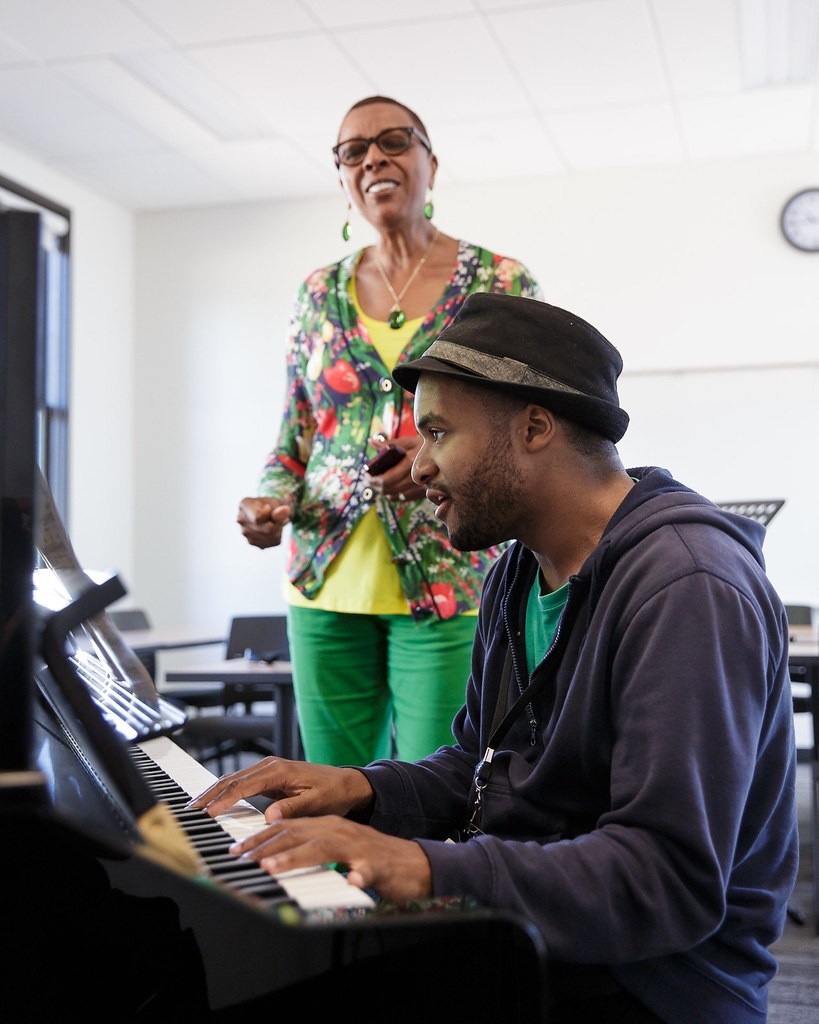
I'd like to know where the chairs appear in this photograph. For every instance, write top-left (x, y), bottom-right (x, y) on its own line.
top-left (784, 606), bottom-right (813, 623)
top-left (186, 615), bottom-right (296, 772)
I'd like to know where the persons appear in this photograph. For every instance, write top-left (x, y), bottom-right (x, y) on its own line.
top-left (234, 94), bottom-right (545, 873)
top-left (184, 291), bottom-right (801, 1023)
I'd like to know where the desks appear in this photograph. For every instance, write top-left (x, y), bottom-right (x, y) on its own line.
top-left (121, 626), bottom-right (228, 685)
top-left (166, 626), bottom-right (819, 938)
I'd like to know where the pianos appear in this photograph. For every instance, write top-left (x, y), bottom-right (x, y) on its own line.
top-left (0, 204), bottom-right (561, 1024)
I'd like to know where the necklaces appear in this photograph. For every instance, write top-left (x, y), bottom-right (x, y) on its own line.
top-left (371, 227), bottom-right (441, 330)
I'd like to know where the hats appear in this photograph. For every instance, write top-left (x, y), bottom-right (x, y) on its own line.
top-left (392, 293), bottom-right (630, 444)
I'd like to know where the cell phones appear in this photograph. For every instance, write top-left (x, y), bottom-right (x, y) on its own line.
top-left (366, 445), bottom-right (408, 478)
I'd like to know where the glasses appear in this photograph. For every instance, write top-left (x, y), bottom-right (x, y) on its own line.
top-left (333, 126), bottom-right (431, 169)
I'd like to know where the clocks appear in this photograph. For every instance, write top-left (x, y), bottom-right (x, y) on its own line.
top-left (779, 187), bottom-right (819, 253)
